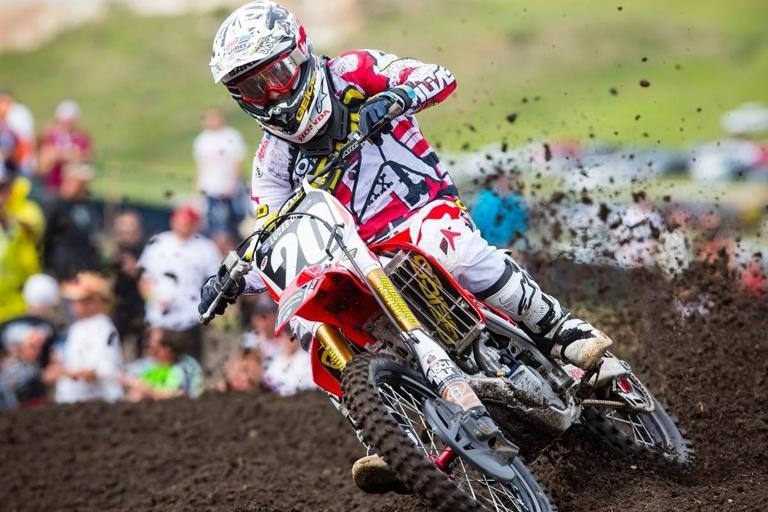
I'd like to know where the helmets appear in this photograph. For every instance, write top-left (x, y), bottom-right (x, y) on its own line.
top-left (208, 0), bottom-right (335, 147)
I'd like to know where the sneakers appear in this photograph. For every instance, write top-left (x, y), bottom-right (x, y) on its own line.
top-left (350, 453), bottom-right (415, 496)
top-left (547, 312), bottom-right (615, 372)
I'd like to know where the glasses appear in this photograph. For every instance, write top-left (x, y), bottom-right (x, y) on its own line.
top-left (234, 28), bottom-right (310, 105)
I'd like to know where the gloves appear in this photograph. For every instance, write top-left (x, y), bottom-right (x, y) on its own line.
top-left (198, 267), bottom-right (247, 321)
top-left (358, 86), bottom-right (413, 147)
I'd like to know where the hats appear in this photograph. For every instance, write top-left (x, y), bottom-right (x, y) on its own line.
top-left (58, 272), bottom-right (114, 302)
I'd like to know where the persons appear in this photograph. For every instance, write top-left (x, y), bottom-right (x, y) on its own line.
top-left (2, 90), bottom-right (317, 410)
top-left (190, 2), bottom-right (611, 496)
top-left (443, 105), bottom-right (768, 312)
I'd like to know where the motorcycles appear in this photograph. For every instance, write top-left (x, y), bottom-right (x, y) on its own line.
top-left (195, 102), bottom-right (714, 511)
